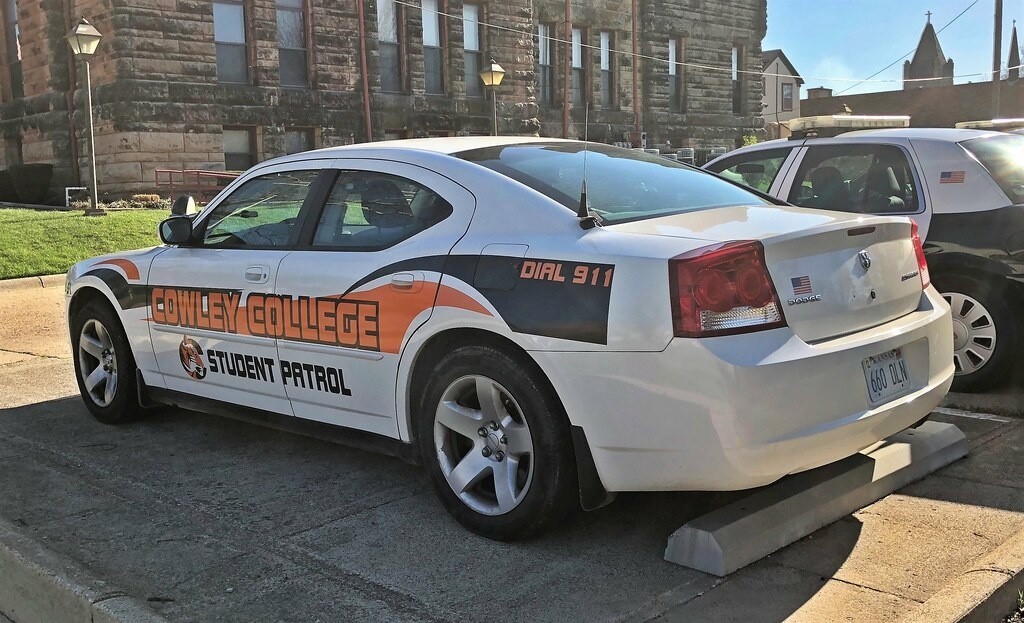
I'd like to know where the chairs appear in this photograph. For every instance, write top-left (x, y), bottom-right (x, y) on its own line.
top-left (344, 178), bottom-right (415, 250)
top-left (863, 163), bottom-right (906, 213)
top-left (806, 165), bottom-right (850, 213)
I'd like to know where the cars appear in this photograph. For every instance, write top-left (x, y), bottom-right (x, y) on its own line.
top-left (60, 133), bottom-right (957, 542)
top-left (695, 115), bottom-right (1023, 398)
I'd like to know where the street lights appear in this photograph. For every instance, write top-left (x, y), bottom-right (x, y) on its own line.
top-left (61, 15), bottom-right (105, 216)
top-left (478, 55), bottom-right (505, 138)
